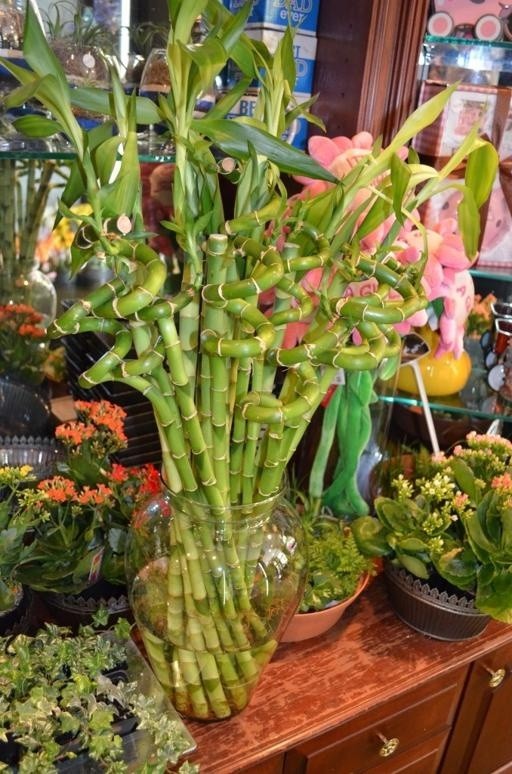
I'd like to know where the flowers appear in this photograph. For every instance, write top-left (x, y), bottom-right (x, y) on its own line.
top-left (1, 459), bottom-right (49, 613)
top-left (26, 397), bottom-right (171, 606)
top-left (256, 138), bottom-right (510, 511)
top-left (359, 428), bottom-right (512, 624)
top-left (0, 304), bottom-right (49, 389)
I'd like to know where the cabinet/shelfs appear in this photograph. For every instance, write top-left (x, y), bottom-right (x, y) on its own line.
top-left (122, 575), bottom-right (512, 774)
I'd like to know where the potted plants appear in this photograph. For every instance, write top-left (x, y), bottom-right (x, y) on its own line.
top-left (129, 19), bottom-right (218, 111)
top-left (0, 0), bottom-right (499, 720)
top-left (246, 489), bottom-right (371, 645)
top-left (0, 613), bottom-right (203, 774)
top-left (35, 4), bottom-right (116, 114)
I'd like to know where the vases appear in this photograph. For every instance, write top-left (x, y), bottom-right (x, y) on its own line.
top-left (304, 327), bottom-right (406, 515)
top-left (0, 378), bottom-right (49, 434)
top-left (383, 557), bottom-right (493, 640)
top-left (31, 584), bottom-right (141, 635)
